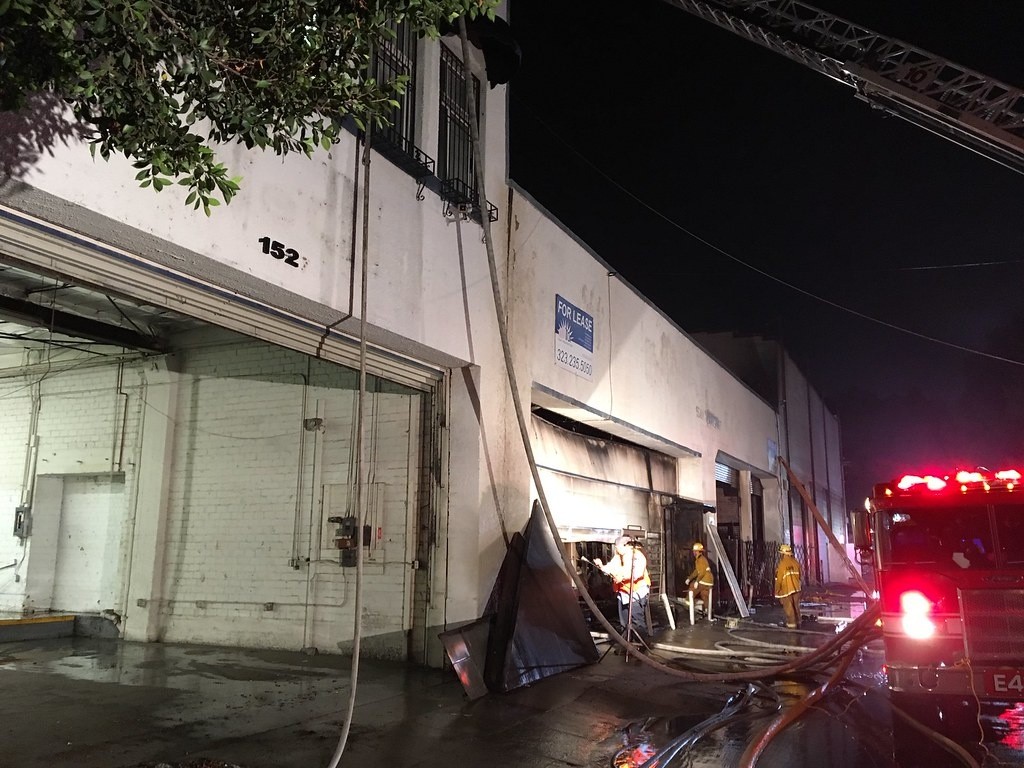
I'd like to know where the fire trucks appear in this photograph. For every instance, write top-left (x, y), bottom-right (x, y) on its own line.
top-left (849, 463), bottom-right (1024, 707)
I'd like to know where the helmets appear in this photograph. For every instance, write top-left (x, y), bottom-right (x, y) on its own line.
top-left (692, 542), bottom-right (706, 552)
top-left (778, 545), bottom-right (795, 556)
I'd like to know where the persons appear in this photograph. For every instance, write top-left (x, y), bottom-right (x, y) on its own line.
top-left (593, 537), bottom-right (654, 655)
top-left (774, 545), bottom-right (802, 630)
top-left (685, 543), bottom-right (713, 619)
